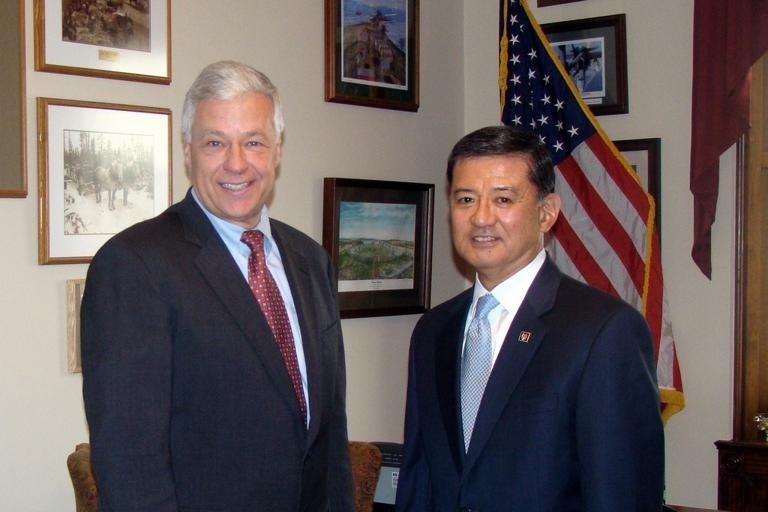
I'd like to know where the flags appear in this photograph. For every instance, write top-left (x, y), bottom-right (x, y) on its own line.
top-left (498, 0), bottom-right (686, 429)
top-left (690, 0), bottom-right (767, 279)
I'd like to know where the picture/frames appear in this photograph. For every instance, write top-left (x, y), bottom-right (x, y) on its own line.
top-left (610, 139), bottom-right (662, 227)
top-left (323, 2), bottom-right (419, 116)
top-left (525, 14), bottom-right (629, 116)
top-left (2, 1), bottom-right (28, 200)
top-left (35, 95), bottom-right (172, 267)
top-left (320, 174), bottom-right (435, 318)
top-left (33, 1), bottom-right (172, 85)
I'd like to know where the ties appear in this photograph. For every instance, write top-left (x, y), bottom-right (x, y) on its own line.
top-left (240, 230), bottom-right (307, 431)
top-left (460, 294), bottom-right (501, 455)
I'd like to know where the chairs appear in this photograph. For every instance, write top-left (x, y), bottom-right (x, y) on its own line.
top-left (71, 439), bottom-right (383, 510)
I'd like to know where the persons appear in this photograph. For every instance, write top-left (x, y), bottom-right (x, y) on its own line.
top-left (79, 60), bottom-right (357, 511)
top-left (393, 126), bottom-right (667, 511)
